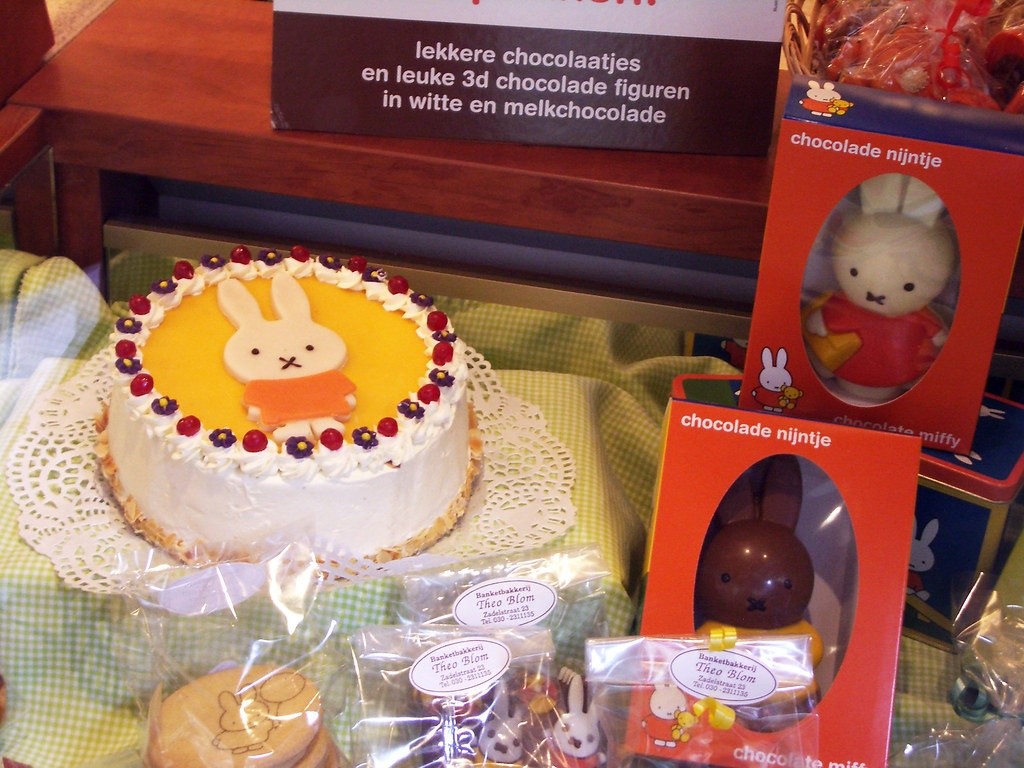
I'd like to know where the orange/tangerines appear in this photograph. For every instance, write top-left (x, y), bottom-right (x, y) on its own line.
top-left (824, 21), bottom-right (976, 108)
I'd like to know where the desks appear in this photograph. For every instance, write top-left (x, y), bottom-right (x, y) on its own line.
top-left (1, 1), bottom-right (1024, 404)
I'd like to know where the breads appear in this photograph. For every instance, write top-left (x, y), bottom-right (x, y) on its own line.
top-left (143, 665), bottom-right (341, 767)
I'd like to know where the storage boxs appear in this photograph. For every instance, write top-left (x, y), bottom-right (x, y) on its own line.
top-left (269, 1), bottom-right (791, 158)
top-left (738, 76), bottom-right (1023, 456)
top-left (899, 393), bottom-right (1024, 656)
top-left (623, 397), bottom-right (921, 768)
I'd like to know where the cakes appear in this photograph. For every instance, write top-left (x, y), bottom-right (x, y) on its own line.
top-left (96, 243), bottom-right (480, 572)
